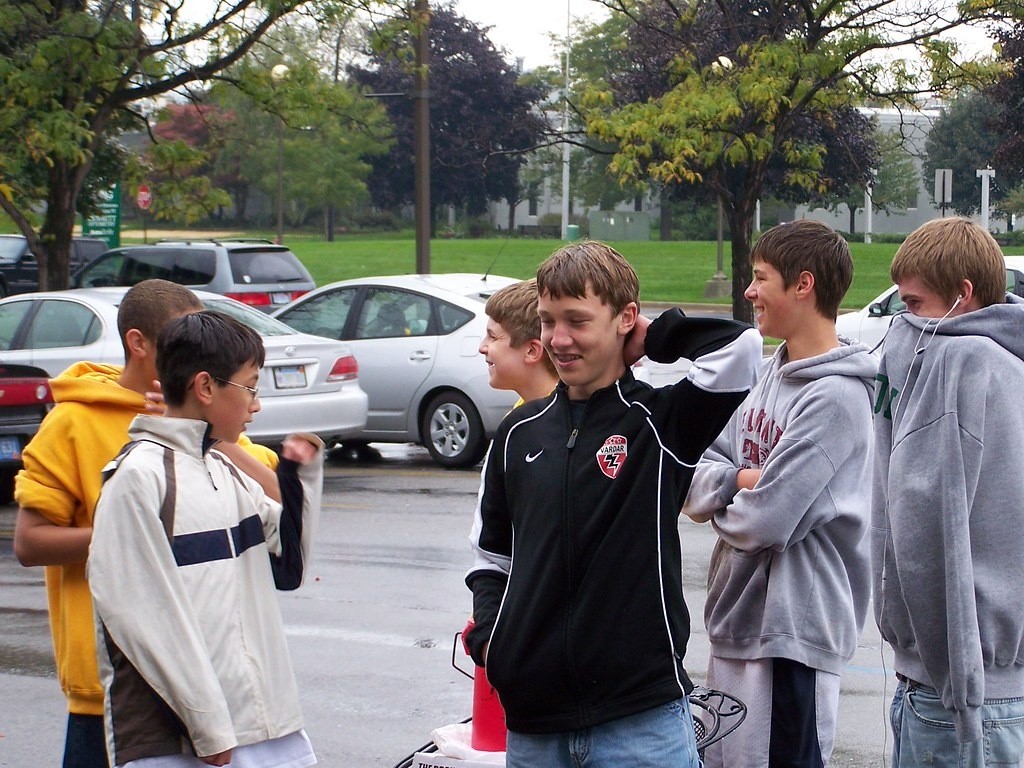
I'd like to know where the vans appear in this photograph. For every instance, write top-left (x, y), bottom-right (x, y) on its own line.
top-left (0, 234), bottom-right (107, 296)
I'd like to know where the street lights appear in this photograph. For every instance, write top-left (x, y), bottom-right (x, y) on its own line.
top-left (269, 64), bottom-right (292, 239)
top-left (710, 54), bottom-right (734, 282)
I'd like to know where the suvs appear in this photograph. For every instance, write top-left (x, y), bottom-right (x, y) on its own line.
top-left (71, 238), bottom-right (316, 314)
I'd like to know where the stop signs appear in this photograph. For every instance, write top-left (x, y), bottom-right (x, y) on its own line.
top-left (137, 185), bottom-right (152, 209)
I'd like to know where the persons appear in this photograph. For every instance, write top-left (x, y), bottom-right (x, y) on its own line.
top-left (680, 220), bottom-right (878, 768)
top-left (86, 313), bottom-right (324, 767)
top-left (464, 238), bottom-right (764, 768)
top-left (13, 279), bottom-right (280, 768)
top-left (479, 278), bottom-right (559, 411)
top-left (869, 220), bottom-right (1024, 768)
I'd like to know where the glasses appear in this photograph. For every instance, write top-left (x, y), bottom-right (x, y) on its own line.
top-left (187, 373), bottom-right (260, 401)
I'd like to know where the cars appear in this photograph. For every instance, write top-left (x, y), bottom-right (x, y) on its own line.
top-left (0, 364), bottom-right (55, 504)
top-left (835, 255), bottom-right (1024, 355)
top-left (0, 285), bottom-right (367, 449)
top-left (270, 236), bottom-right (647, 469)
